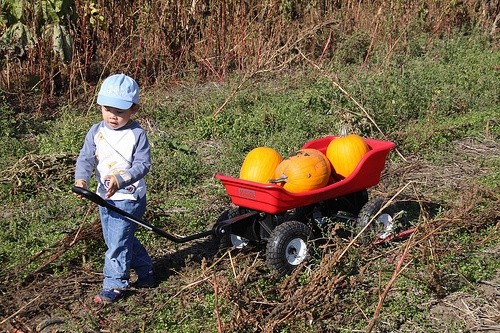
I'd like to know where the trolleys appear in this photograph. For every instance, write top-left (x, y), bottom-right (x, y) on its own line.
top-left (73, 133), bottom-right (397, 278)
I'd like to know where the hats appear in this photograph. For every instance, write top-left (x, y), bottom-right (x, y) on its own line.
top-left (97, 73), bottom-right (140, 110)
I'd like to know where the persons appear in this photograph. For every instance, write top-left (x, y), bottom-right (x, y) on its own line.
top-left (74, 73), bottom-right (155, 304)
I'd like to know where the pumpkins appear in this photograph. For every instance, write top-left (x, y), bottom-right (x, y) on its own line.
top-left (267, 148), bottom-right (331, 193)
top-left (325, 134), bottom-right (371, 181)
top-left (239, 146), bottom-right (283, 185)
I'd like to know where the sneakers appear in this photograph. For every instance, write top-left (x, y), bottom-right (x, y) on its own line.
top-left (131, 272), bottom-right (156, 288)
top-left (94, 287), bottom-right (126, 303)
